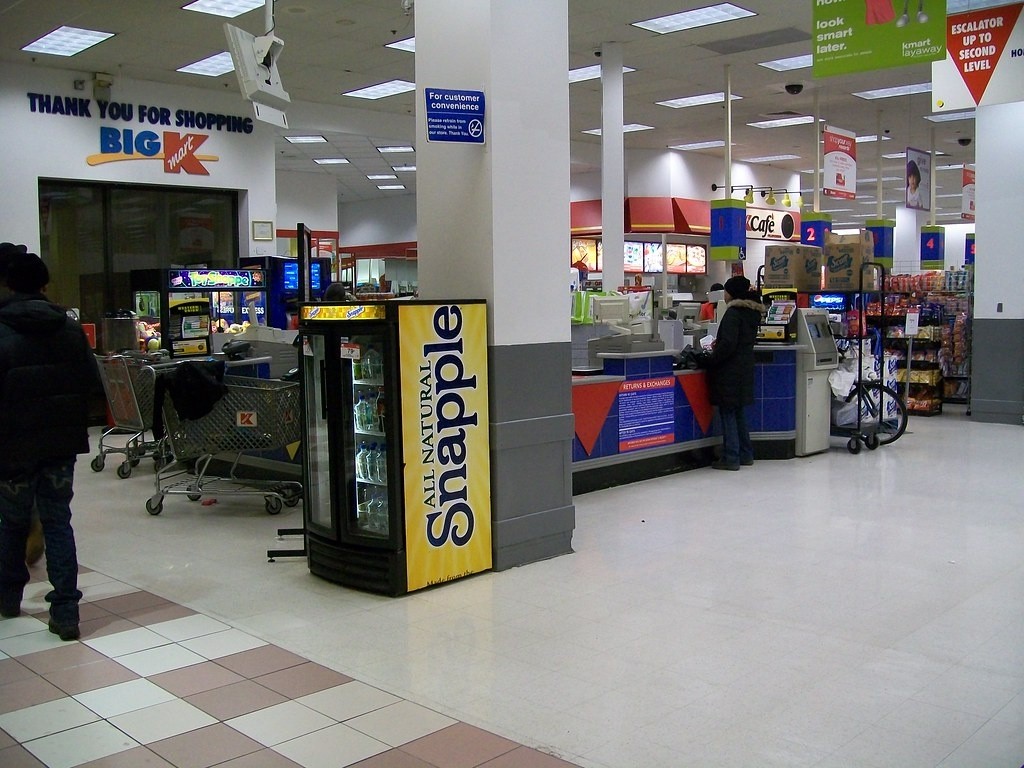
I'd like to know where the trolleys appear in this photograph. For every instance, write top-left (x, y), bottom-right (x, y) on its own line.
top-left (92, 353), bottom-right (192, 478)
top-left (145, 360), bottom-right (303, 516)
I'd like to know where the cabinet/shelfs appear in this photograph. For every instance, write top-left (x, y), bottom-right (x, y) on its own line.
top-left (942, 268), bottom-right (975, 405)
top-left (863, 272), bottom-right (943, 416)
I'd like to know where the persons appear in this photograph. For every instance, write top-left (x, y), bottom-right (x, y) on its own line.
top-left (324, 283), bottom-right (358, 302)
top-left (906, 158), bottom-right (924, 209)
top-left (693, 275), bottom-right (766, 470)
top-left (0, 242), bottom-right (118, 641)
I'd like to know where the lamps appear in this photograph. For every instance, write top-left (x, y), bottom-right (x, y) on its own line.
top-left (730, 185), bottom-right (776, 206)
top-left (760, 190), bottom-right (804, 210)
top-left (711, 183), bottom-right (755, 204)
top-left (745, 188), bottom-right (791, 207)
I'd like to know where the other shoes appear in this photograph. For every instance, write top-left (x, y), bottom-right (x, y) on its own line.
top-left (0, 597), bottom-right (22, 617)
top-left (740, 458), bottom-right (754, 466)
top-left (25, 515), bottom-right (46, 566)
top-left (48, 618), bottom-right (80, 641)
top-left (711, 458), bottom-right (740, 471)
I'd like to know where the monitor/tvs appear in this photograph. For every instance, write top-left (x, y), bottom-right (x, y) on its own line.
top-left (285, 262), bottom-right (321, 290)
top-left (223, 22), bottom-right (290, 112)
top-left (677, 302), bottom-right (701, 330)
top-left (593, 296), bottom-right (630, 325)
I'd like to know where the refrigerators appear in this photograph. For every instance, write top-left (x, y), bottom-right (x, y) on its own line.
top-left (298, 299), bottom-right (493, 598)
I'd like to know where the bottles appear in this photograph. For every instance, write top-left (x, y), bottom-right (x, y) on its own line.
top-left (357, 442), bottom-right (386, 481)
top-left (355, 387), bottom-right (385, 432)
top-left (354, 346), bottom-right (380, 378)
top-left (361, 484), bottom-right (388, 531)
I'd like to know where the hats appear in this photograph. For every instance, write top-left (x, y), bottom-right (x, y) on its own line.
top-left (323, 283), bottom-right (345, 301)
top-left (0, 242), bottom-right (27, 267)
top-left (6, 253), bottom-right (49, 288)
top-left (724, 275), bottom-right (751, 300)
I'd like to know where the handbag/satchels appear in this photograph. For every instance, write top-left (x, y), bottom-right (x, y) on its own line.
top-left (678, 344), bottom-right (713, 370)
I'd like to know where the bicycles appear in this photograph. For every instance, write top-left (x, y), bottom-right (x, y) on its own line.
top-left (838, 348), bottom-right (908, 447)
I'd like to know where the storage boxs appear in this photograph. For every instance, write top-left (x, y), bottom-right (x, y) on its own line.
top-left (823, 230), bottom-right (876, 289)
top-left (764, 245), bottom-right (821, 289)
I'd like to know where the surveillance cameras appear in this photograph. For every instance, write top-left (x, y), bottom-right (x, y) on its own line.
top-left (595, 51), bottom-right (600, 57)
top-left (885, 130), bottom-right (889, 133)
top-left (785, 85), bottom-right (803, 94)
top-left (958, 139), bottom-right (971, 147)
